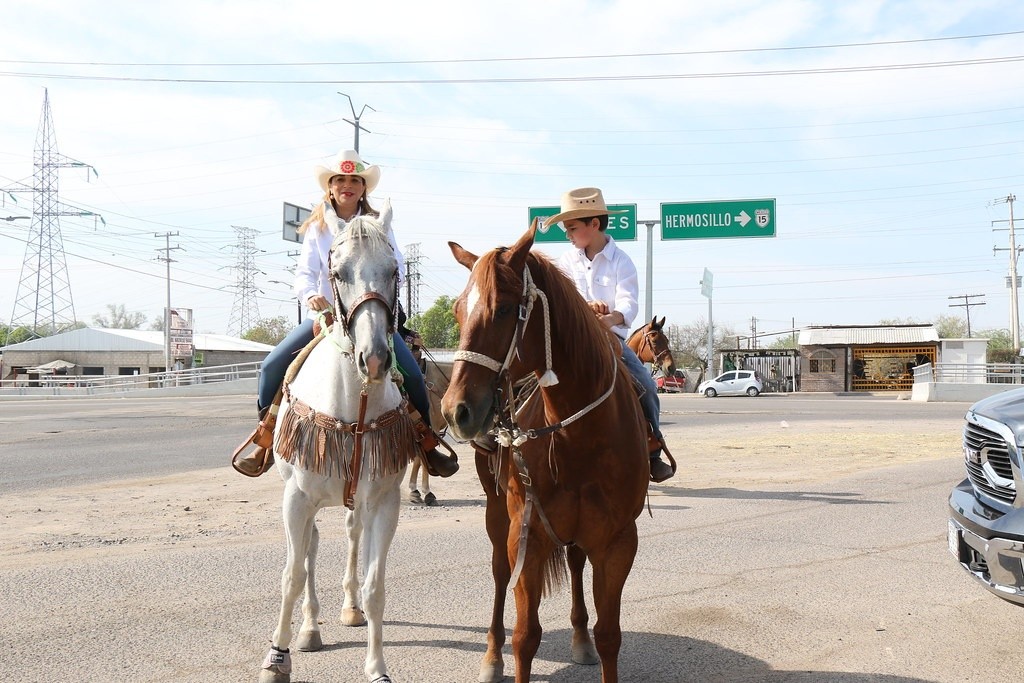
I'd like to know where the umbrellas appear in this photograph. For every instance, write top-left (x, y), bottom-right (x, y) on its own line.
top-left (38, 359), bottom-right (79, 369)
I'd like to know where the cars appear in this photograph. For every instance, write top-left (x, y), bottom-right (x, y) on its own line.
top-left (652, 370), bottom-right (686, 393)
top-left (947, 385), bottom-right (1024, 605)
top-left (699, 370), bottom-right (764, 397)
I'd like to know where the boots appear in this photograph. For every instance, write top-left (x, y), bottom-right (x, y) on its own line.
top-left (235, 399), bottom-right (275, 473)
top-left (417, 404), bottom-right (458, 477)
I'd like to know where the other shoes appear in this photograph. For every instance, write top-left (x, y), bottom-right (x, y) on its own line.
top-left (650, 455), bottom-right (672, 479)
top-left (474, 430), bottom-right (497, 451)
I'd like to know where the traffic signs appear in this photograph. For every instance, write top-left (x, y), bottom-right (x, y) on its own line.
top-left (660, 197), bottom-right (779, 241)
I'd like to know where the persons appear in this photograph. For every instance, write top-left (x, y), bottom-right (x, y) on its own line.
top-left (235, 148), bottom-right (459, 477)
top-left (543, 187), bottom-right (674, 481)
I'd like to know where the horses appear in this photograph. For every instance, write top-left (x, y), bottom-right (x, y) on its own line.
top-left (623, 316), bottom-right (676, 378)
top-left (441, 215), bottom-right (650, 683)
top-left (408, 361), bottom-right (457, 506)
top-left (257, 197), bottom-right (408, 683)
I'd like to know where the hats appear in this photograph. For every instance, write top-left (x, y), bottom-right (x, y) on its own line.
top-left (319, 151), bottom-right (380, 195)
top-left (544, 188), bottom-right (630, 230)
